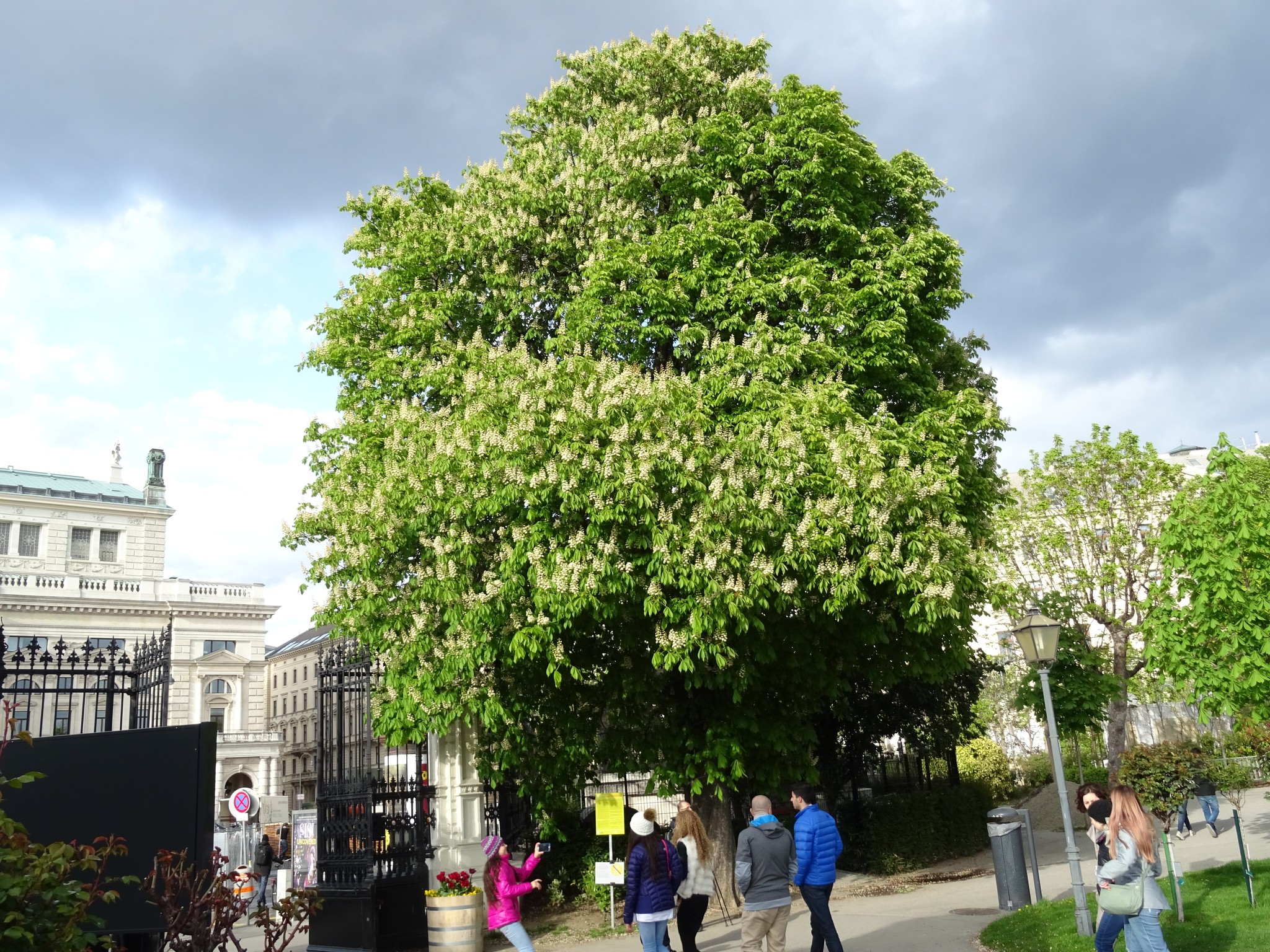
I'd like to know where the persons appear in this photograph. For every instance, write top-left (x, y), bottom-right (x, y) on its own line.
top-left (307, 852), bottom-right (316, 886)
top-left (790, 785), bottom-right (844, 952)
top-left (1075, 782), bottom-right (1171, 952)
top-left (254, 834), bottom-right (286, 907)
top-left (1176, 748), bottom-right (1219, 840)
top-left (480, 833), bottom-right (544, 951)
top-left (623, 800), bottom-right (714, 952)
top-left (735, 794), bottom-right (799, 952)
top-left (277, 823), bottom-right (289, 858)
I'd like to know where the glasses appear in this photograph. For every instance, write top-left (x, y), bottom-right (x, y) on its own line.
top-left (679, 806), bottom-right (691, 811)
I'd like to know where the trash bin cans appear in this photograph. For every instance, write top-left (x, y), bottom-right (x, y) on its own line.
top-left (986, 807), bottom-right (1042, 912)
top-left (234, 864), bottom-right (254, 900)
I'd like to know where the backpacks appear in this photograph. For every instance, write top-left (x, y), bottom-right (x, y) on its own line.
top-left (255, 843), bottom-right (270, 865)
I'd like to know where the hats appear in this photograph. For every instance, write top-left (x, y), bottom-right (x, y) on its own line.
top-left (481, 835), bottom-right (503, 860)
top-left (630, 808), bottom-right (657, 836)
top-left (1087, 799), bottom-right (1112, 823)
top-left (262, 834), bottom-right (269, 841)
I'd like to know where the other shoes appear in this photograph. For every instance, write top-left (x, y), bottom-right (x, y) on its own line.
top-left (257, 901), bottom-right (261, 908)
top-left (261, 902), bottom-right (267, 906)
top-left (284, 858), bottom-right (290, 861)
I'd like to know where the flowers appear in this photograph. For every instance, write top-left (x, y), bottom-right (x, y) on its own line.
top-left (422, 868), bottom-right (481, 897)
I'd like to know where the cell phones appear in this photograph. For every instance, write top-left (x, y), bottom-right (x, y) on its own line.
top-left (538, 843), bottom-right (551, 852)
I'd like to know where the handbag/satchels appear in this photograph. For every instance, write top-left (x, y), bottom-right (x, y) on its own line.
top-left (673, 898), bottom-right (681, 918)
top-left (1098, 883), bottom-right (1143, 915)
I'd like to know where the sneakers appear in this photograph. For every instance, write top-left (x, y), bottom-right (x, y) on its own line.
top-left (1189, 829), bottom-right (1195, 836)
top-left (1206, 821), bottom-right (1218, 838)
top-left (1176, 831), bottom-right (1185, 840)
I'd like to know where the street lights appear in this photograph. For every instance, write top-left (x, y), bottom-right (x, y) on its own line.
top-left (1012, 607), bottom-right (1095, 940)
top-left (299, 753), bottom-right (315, 810)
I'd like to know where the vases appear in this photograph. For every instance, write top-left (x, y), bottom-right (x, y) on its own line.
top-left (426, 891), bottom-right (483, 952)
